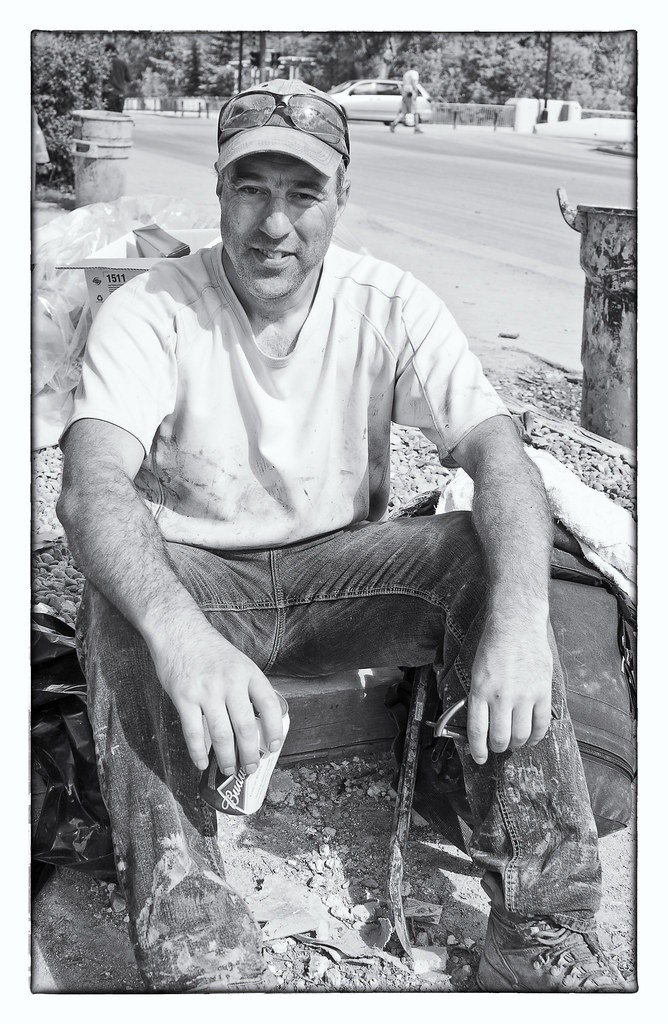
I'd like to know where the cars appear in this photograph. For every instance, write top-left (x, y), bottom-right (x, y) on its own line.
top-left (324, 79), bottom-right (434, 127)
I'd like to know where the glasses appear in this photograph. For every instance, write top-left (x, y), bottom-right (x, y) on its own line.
top-left (216, 90), bottom-right (351, 155)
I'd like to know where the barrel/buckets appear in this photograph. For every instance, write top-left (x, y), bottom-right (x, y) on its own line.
top-left (69, 110), bottom-right (134, 208)
top-left (553, 185), bottom-right (637, 447)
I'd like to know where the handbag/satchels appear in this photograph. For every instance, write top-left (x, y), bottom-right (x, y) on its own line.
top-left (405, 112), bottom-right (416, 126)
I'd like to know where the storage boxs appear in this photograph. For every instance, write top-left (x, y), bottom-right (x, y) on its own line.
top-left (54, 227), bottom-right (222, 321)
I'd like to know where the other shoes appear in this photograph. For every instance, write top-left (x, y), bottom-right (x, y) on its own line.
top-left (474, 898), bottom-right (632, 993)
top-left (414, 128), bottom-right (424, 134)
top-left (390, 122), bottom-right (396, 132)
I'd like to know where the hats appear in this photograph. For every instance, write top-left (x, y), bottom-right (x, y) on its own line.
top-left (216, 78), bottom-right (351, 180)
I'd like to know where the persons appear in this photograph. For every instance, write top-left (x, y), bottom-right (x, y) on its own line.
top-left (53, 79), bottom-right (627, 994)
top-left (100, 42), bottom-right (132, 112)
top-left (390, 64), bottom-right (424, 133)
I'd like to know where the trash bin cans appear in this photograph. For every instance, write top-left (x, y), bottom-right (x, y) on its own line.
top-left (554, 187), bottom-right (637, 450)
top-left (66, 109), bottom-right (136, 211)
